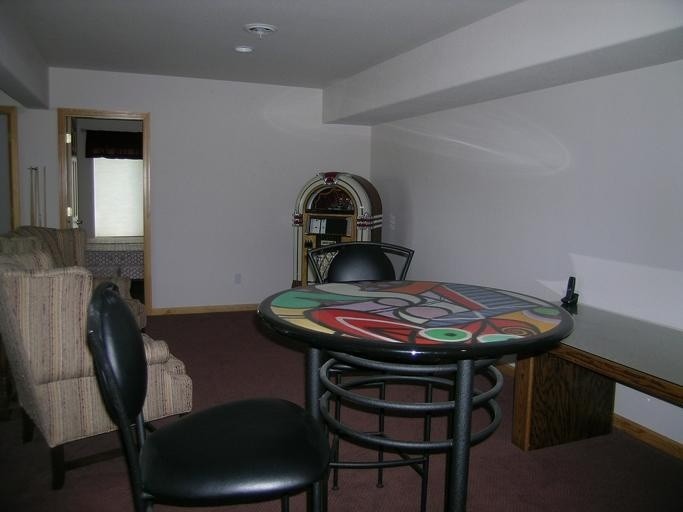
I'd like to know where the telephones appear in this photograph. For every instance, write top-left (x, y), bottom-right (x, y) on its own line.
top-left (560, 277), bottom-right (579, 305)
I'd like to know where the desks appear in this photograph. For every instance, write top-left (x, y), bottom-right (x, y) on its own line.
top-left (258, 280), bottom-right (576, 511)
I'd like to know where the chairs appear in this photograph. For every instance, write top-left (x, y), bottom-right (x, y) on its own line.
top-left (87, 283), bottom-right (331, 512)
top-left (306, 242), bottom-right (433, 490)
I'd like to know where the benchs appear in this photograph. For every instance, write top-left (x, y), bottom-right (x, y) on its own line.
top-left (511, 303), bottom-right (682, 452)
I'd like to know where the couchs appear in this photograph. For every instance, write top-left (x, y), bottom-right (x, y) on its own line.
top-left (18, 227), bottom-right (148, 335)
top-left (0, 237), bottom-right (194, 491)
